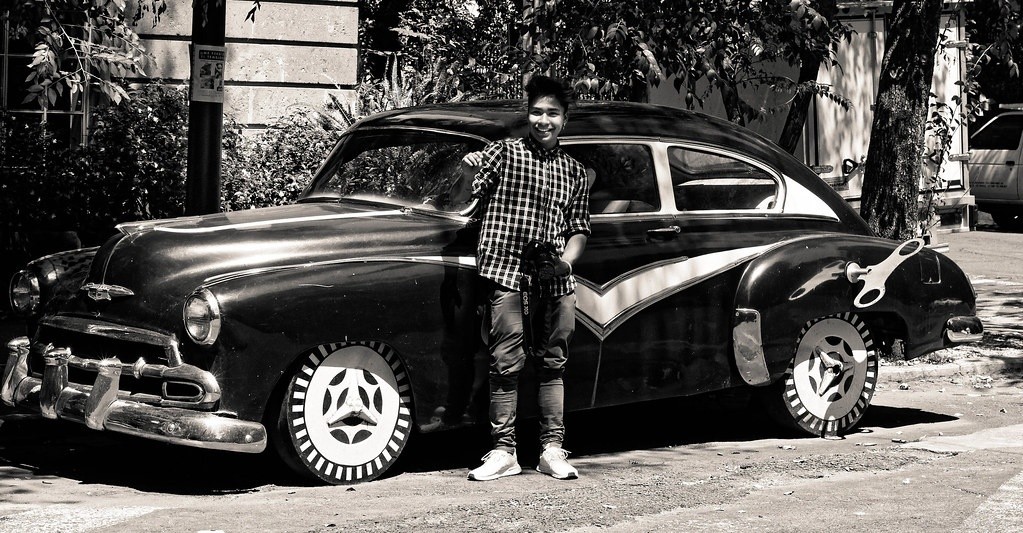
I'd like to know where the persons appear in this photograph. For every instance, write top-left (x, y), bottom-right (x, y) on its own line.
top-left (449, 74), bottom-right (591, 480)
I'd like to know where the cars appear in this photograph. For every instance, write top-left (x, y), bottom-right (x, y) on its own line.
top-left (0, 97), bottom-right (986, 495)
top-left (968, 102), bottom-right (1023, 226)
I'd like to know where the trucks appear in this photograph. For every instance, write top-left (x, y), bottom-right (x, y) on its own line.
top-left (635, 0), bottom-right (976, 254)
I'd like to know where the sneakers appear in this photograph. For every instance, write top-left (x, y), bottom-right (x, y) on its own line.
top-left (467, 448), bottom-right (522, 481)
top-left (536, 443), bottom-right (578, 479)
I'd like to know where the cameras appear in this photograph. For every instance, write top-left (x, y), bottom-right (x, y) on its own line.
top-left (521, 236), bottom-right (560, 281)
